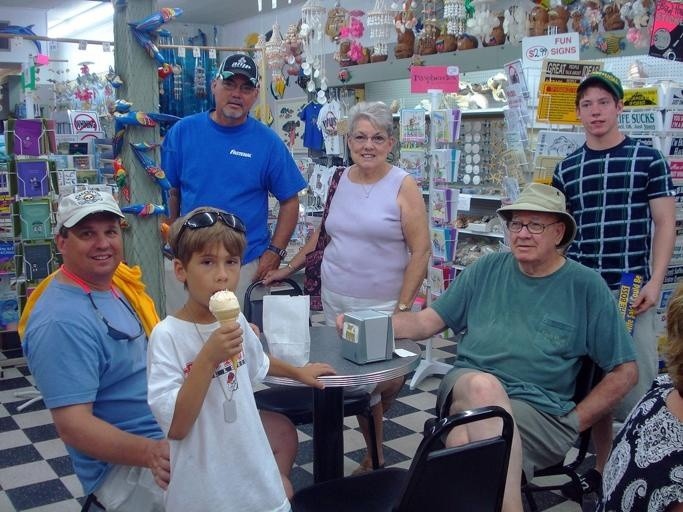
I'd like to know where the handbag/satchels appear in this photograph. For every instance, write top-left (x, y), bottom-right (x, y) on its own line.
top-left (303, 249), bottom-right (324, 312)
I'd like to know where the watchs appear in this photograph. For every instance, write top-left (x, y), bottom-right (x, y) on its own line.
top-left (267, 243), bottom-right (287, 259)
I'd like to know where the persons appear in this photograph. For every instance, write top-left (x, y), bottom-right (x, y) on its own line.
top-left (156, 52), bottom-right (309, 315)
top-left (337, 179), bottom-right (640, 509)
top-left (262, 99), bottom-right (432, 477)
top-left (594, 275), bottom-right (682, 511)
top-left (548, 68), bottom-right (677, 489)
top-left (146, 205), bottom-right (337, 512)
top-left (18, 188), bottom-right (298, 512)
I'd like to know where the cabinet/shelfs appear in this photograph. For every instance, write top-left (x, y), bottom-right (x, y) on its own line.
top-left (454, 194), bottom-right (509, 272)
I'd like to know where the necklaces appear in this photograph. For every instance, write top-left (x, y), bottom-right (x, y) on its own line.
top-left (181, 301), bottom-right (238, 423)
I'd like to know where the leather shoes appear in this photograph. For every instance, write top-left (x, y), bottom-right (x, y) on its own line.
top-left (559, 466), bottom-right (604, 499)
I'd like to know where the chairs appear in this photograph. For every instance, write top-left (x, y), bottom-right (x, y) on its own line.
top-left (242, 276), bottom-right (382, 472)
top-left (423, 359), bottom-right (601, 512)
top-left (80, 494), bottom-right (105, 512)
top-left (289, 407), bottom-right (514, 512)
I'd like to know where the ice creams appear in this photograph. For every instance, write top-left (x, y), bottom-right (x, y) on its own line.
top-left (209, 288), bottom-right (240, 363)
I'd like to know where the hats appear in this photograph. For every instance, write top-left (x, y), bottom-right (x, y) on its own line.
top-left (55, 189), bottom-right (125, 237)
top-left (496, 183), bottom-right (578, 250)
top-left (215, 53), bottom-right (260, 88)
top-left (168, 205), bottom-right (227, 256)
top-left (577, 71), bottom-right (624, 99)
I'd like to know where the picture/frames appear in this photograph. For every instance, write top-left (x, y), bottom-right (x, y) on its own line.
top-left (274, 96), bottom-right (308, 153)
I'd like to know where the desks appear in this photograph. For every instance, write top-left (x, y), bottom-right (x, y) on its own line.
top-left (260, 325), bottom-right (426, 487)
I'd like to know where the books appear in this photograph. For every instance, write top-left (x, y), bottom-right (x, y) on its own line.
top-left (0, 111), bottom-right (116, 341)
top-left (396, 104), bottom-right (461, 296)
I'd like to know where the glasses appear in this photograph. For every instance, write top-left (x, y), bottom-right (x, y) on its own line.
top-left (222, 79), bottom-right (254, 94)
top-left (87, 293), bottom-right (145, 343)
top-left (354, 135), bottom-right (387, 144)
top-left (506, 220), bottom-right (563, 235)
top-left (174, 211), bottom-right (247, 247)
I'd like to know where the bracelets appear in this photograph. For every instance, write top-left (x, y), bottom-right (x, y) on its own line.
top-left (286, 263), bottom-right (297, 273)
top-left (397, 302), bottom-right (411, 311)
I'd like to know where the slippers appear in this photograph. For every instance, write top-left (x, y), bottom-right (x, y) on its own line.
top-left (351, 459), bottom-right (386, 477)
